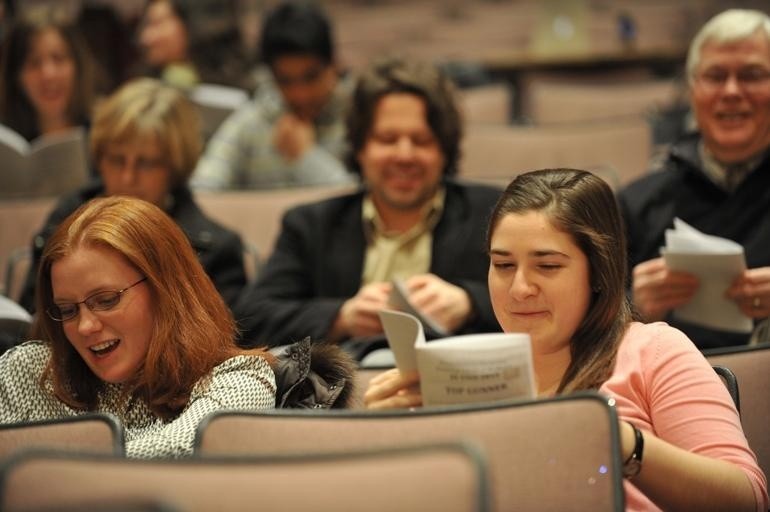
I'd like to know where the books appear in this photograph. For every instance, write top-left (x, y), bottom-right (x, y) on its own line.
top-left (376, 309), bottom-right (538, 406)
top-left (0, 123), bottom-right (88, 190)
top-left (657, 219), bottom-right (753, 336)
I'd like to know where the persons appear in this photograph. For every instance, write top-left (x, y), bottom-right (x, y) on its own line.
top-left (615, 8), bottom-right (770, 352)
top-left (0, 1), bottom-right (103, 188)
top-left (179, 3), bottom-right (355, 191)
top-left (0, 192), bottom-right (280, 461)
top-left (17, 81), bottom-right (246, 308)
top-left (361, 168), bottom-right (769, 511)
top-left (223, 55), bottom-right (508, 368)
top-left (111, 1), bottom-right (209, 89)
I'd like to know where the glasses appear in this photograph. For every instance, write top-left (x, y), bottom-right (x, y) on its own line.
top-left (44, 278), bottom-right (145, 323)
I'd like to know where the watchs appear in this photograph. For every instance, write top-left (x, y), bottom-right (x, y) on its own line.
top-left (615, 416), bottom-right (647, 484)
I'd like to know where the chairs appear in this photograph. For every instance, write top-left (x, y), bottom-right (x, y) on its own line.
top-left (1, 2), bottom-right (768, 315)
top-left (699, 344), bottom-right (765, 477)
top-left (2, 412), bottom-right (126, 473)
top-left (4, 444), bottom-right (490, 512)
top-left (191, 389), bottom-right (627, 510)
